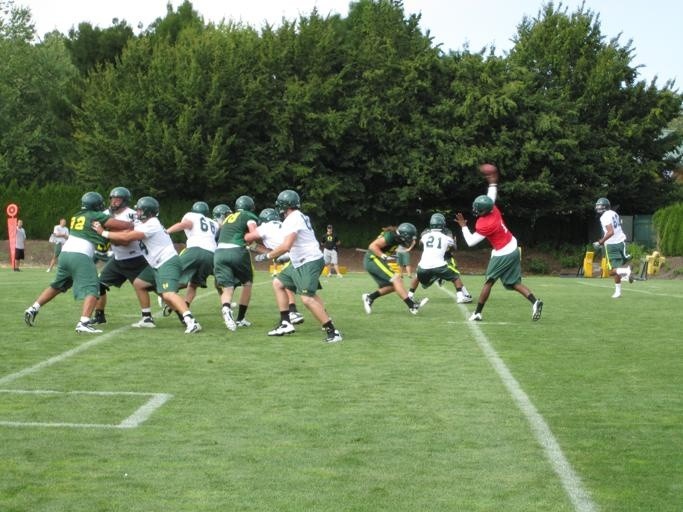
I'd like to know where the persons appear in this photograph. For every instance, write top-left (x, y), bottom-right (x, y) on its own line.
top-left (24, 187), bottom-right (203, 335)
top-left (591, 195), bottom-right (632, 302)
top-left (360, 213), bottom-right (472, 316)
top-left (455, 165), bottom-right (543, 322)
top-left (14, 220), bottom-right (26, 272)
top-left (161, 193), bottom-right (342, 344)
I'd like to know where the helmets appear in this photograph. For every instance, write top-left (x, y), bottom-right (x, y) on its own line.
top-left (473, 195), bottom-right (493, 217)
top-left (595, 198), bottom-right (611, 212)
top-left (429, 212), bottom-right (446, 230)
top-left (396, 222), bottom-right (417, 248)
top-left (81, 187), bottom-right (303, 221)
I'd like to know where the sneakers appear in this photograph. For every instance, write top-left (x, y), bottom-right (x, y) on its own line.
top-left (627, 265), bottom-right (633, 283)
top-left (362, 283), bottom-right (543, 322)
top-left (612, 292), bottom-right (622, 298)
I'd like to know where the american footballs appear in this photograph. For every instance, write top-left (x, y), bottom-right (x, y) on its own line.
top-left (480, 164), bottom-right (495, 173)
